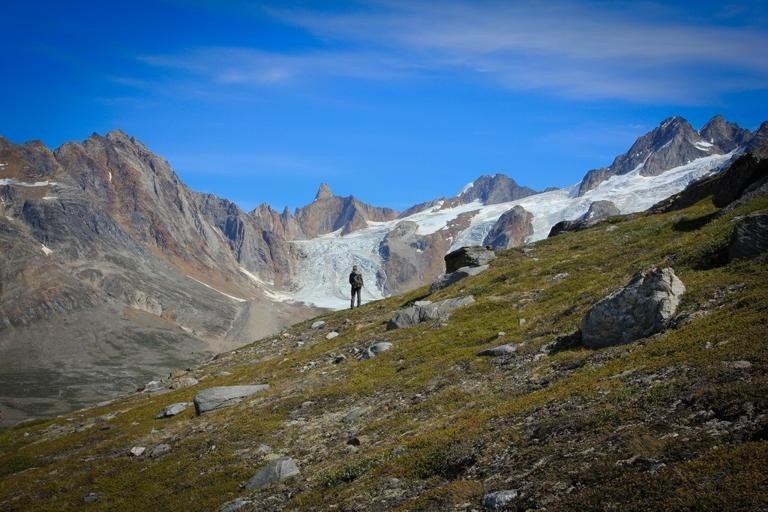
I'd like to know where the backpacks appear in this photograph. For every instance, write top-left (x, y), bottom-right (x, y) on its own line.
top-left (351, 275), bottom-right (363, 290)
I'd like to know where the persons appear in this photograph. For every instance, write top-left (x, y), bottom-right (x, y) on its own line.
top-left (349, 266), bottom-right (363, 309)
